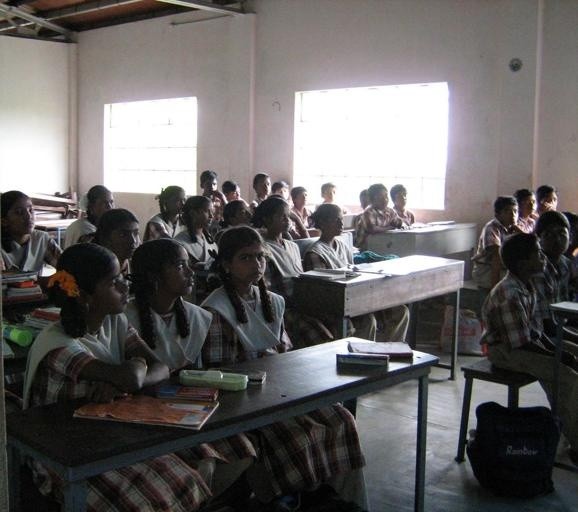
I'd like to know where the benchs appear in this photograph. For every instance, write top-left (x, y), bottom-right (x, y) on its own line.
top-left (454, 358), bottom-right (542, 464)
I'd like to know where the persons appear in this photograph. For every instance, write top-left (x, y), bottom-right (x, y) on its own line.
top-left (22, 243), bottom-right (212, 511)
top-left (303, 204), bottom-right (409, 342)
top-left (260, 198), bottom-right (355, 349)
top-left (390, 184), bottom-right (414, 226)
top-left (124, 239), bottom-right (255, 510)
top-left (201, 225), bottom-right (368, 512)
top-left (351, 184), bottom-right (402, 249)
top-left (0, 191), bottom-right (62, 277)
top-left (471, 184), bottom-right (577, 451)
top-left (143, 168), bottom-right (336, 306)
top-left (64, 185), bottom-right (139, 295)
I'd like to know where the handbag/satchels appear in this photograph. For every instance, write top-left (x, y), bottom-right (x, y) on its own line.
top-left (465, 401), bottom-right (559, 498)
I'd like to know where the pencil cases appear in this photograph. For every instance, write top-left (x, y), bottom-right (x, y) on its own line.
top-left (336, 354), bottom-right (389, 366)
top-left (180, 370), bottom-right (247, 392)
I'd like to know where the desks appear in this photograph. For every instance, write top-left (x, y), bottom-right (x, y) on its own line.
top-left (293, 254), bottom-right (464, 423)
top-left (1, 338), bottom-right (443, 510)
top-left (2, 223), bottom-right (481, 380)
